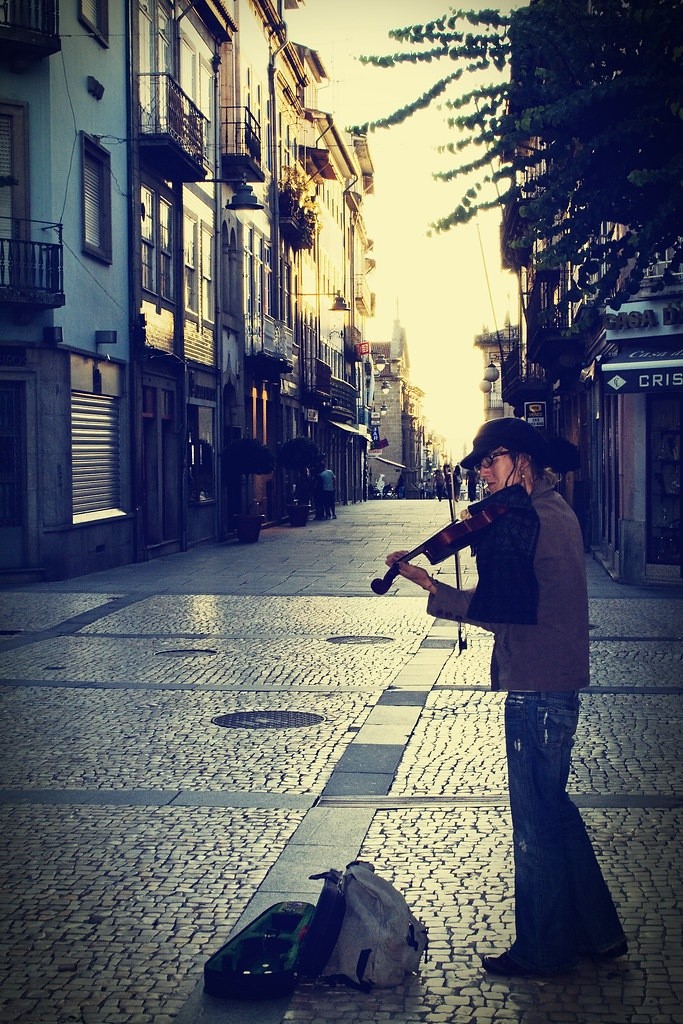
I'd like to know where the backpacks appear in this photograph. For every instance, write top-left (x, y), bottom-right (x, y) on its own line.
top-left (338, 860), bottom-right (429, 993)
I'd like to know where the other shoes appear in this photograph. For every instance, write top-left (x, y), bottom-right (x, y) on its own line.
top-left (599, 940), bottom-right (628, 956)
top-left (483, 950), bottom-right (540, 976)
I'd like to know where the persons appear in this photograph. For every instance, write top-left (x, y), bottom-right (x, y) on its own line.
top-left (312, 462), bottom-right (337, 521)
top-left (465, 467), bottom-right (478, 502)
top-left (434, 468), bottom-right (444, 502)
top-left (396, 474), bottom-right (405, 500)
top-left (453, 465), bottom-right (463, 502)
top-left (383, 416), bottom-right (629, 975)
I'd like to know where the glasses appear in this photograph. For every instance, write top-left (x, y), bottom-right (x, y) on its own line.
top-left (474, 451), bottom-right (510, 472)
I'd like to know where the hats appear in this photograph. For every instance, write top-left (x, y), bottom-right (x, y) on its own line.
top-left (460, 418), bottom-right (550, 468)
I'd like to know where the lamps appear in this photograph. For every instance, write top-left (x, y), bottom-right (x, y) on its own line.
top-left (297, 290), bottom-right (350, 321)
top-left (484, 350), bottom-right (518, 381)
top-left (373, 401), bottom-right (388, 416)
top-left (375, 379), bottom-right (392, 394)
top-left (370, 350), bottom-right (389, 372)
top-left (479, 379), bottom-right (492, 393)
top-left (195, 172), bottom-right (264, 225)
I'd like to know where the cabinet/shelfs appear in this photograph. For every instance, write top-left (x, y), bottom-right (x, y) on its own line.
top-left (649, 429), bottom-right (683, 565)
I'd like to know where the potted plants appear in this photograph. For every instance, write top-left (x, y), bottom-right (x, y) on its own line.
top-left (219, 438), bottom-right (277, 544)
top-left (278, 433), bottom-right (327, 528)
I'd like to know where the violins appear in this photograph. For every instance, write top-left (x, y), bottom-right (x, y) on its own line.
top-left (369, 484), bottom-right (532, 597)
